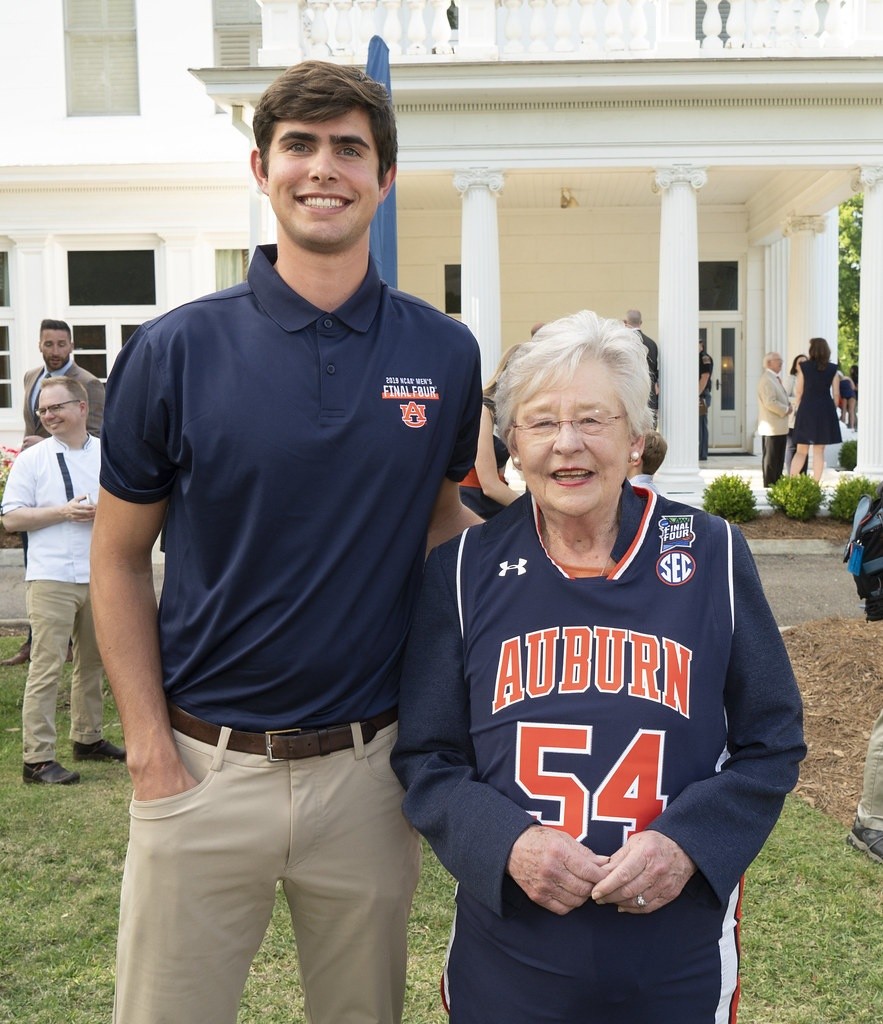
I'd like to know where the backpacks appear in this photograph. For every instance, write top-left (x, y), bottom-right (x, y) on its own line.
top-left (843, 491), bottom-right (883, 623)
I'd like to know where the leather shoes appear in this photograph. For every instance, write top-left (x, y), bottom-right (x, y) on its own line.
top-left (23, 762), bottom-right (80, 785)
top-left (66, 646), bottom-right (75, 662)
top-left (73, 739), bottom-right (126, 761)
top-left (1, 643), bottom-right (30, 666)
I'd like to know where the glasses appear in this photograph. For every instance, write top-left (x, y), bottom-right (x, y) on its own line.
top-left (36, 399), bottom-right (82, 418)
top-left (515, 411), bottom-right (627, 441)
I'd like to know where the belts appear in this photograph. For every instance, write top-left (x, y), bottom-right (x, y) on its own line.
top-left (165, 700), bottom-right (398, 762)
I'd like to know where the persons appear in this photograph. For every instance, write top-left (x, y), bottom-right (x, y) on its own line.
top-left (837, 366), bottom-right (858, 429)
top-left (0, 319), bottom-right (104, 666)
top-left (842, 482), bottom-right (883, 863)
top-left (461, 323), bottom-right (543, 518)
top-left (625, 309), bottom-right (658, 415)
top-left (759, 351), bottom-right (808, 488)
top-left (0, 379), bottom-right (127, 783)
top-left (88, 61), bottom-right (483, 1024)
top-left (699, 339), bottom-right (714, 461)
top-left (391, 310), bottom-right (807, 1024)
top-left (627, 432), bottom-right (667, 493)
top-left (790, 336), bottom-right (839, 482)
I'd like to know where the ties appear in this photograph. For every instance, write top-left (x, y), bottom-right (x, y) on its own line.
top-left (33, 373), bottom-right (51, 424)
top-left (778, 377), bottom-right (788, 396)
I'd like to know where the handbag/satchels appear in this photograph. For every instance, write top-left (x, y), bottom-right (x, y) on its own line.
top-left (699, 400), bottom-right (708, 416)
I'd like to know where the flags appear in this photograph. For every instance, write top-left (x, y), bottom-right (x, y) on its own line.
top-left (365, 36), bottom-right (396, 285)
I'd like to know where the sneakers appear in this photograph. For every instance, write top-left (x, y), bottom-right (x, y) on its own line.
top-left (847, 817), bottom-right (883, 864)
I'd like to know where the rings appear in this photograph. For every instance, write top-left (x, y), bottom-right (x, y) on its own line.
top-left (637, 894), bottom-right (648, 906)
